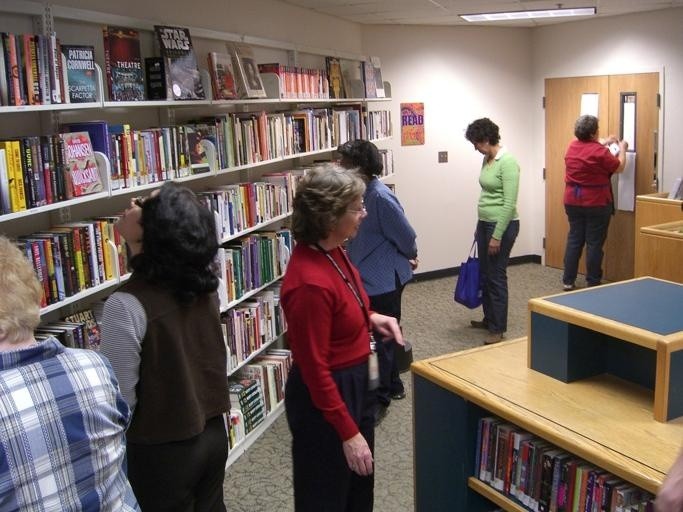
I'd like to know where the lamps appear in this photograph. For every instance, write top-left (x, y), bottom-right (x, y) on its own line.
top-left (457, 7), bottom-right (598, 23)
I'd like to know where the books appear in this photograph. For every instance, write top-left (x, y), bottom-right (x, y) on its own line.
top-left (15, 213), bottom-right (132, 308)
top-left (219, 280), bottom-right (290, 375)
top-left (155, 25), bottom-right (207, 100)
top-left (259, 63), bottom-right (330, 99)
top-left (379, 149), bottom-right (396, 179)
top-left (213, 228), bottom-right (293, 309)
top-left (476, 416), bottom-right (657, 512)
top-left (62, 45), bottom-right (97, 102)
top-left (214, 103), bottom-right (393, 171)
top-left (228, 42), bottom-right (268, 98)
top-left (33, 308), bottom-right (102, 349)
top-left (221, 347), bottom-right (294, 450)
top-left (1, 135), bottom-right (67, 216)
top-left (1, 30), bottom-right (63, 106)
top-left (326, 56), bottom-right (347, 99)
top-left (102, 24), bottom-right (146, 100)
top-left (61, 116), bottom-right (214, 190)
top-left (194, 165), bottom-right (311, 240)
top-left (209, 52), bottom-right (239, 99)
top-left (60, 132), bottom-right (103, 197)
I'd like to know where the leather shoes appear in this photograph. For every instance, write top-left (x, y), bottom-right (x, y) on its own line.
top-left (470, 319), bottom-right (487, 330)
top-left (484, 330), bottom-right (504, 346)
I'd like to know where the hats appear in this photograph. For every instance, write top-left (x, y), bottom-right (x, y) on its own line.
top-left (337, 140), bottom-right (383, 175)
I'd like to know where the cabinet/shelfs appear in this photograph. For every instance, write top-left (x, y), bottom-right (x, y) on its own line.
top-left (0, 63), bottom-right (403, 474)
top-left (408, 274), bottom-right (683, 512)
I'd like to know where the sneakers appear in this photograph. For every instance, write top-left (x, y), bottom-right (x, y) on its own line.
top-left (387, 377), bottom-right (404, 399)
top-left (562, 283), bottom-right (574, 290)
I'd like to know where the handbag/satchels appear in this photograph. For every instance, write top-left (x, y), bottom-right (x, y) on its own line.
top-left (454, 239), bottom-right (483, 309)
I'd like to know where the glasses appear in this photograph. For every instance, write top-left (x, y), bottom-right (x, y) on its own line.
top-left (344, 201), bottom-right (367, 215)
top-left (134, 194), bottom-right (144, 208)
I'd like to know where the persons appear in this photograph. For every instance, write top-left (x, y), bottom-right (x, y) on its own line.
top-left (653, 445), bottom-right (678, 510)
top-left (1, 238), bottom-right (141, 512)
top-left (466, 117), bottom-right (521, 345)
top-left (561, 115), bottom-right (628, 288)
top-left (340, 140), bottom-right (419, 399)
top-left (99, 181), bottom-right (232, 512)
top-left (279, 164), bottom-right (406, 512)
